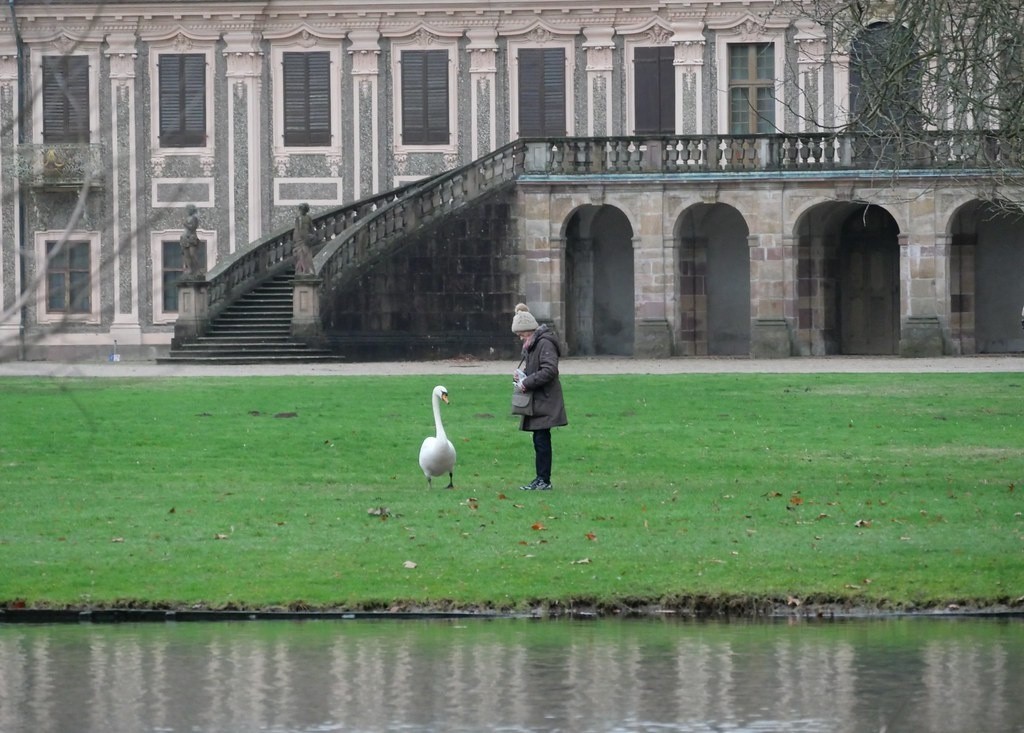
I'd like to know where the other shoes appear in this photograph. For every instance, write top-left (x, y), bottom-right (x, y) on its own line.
top-left (519, 477), bottom-right (554, 491)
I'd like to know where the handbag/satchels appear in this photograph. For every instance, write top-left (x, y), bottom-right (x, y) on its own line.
top-left (511, 392), bottom-right (533, 417)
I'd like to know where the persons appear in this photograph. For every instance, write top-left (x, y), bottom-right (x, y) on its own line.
top-left (179, 204), bottom-right (205, 281)
top-left (512, 302), bottom-right (568, 490)
top-left (293, 202), bottom-right (316, 275)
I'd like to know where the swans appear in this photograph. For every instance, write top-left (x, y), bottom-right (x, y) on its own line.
top-left (419, 386), bottom-right (457, 491)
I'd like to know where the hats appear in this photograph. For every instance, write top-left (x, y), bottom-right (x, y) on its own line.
top-left (511, 303), bottom-right (539, 333)
